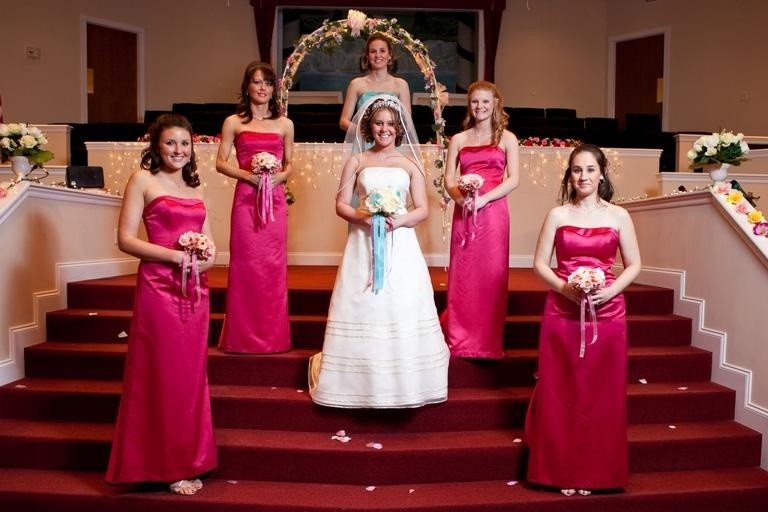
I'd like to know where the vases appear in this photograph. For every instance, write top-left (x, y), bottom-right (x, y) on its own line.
top-left (8, 155), bottom-right (35, 183)
top-left (708, 163), bottom-right (729, 187)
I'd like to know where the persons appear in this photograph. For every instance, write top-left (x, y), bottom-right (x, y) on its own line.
top-left (305, 94), bottom-right (450, 410)
top-left (105, 113), bottom-right (217, 494)
top-left (441, 80), bottom-right (521, 360)
top-left (216, 60), bottom-right (294, 355)
top-left (338, 34), bottom-right (412, 152)
top-left (526, 146), bottom-right (642, 497)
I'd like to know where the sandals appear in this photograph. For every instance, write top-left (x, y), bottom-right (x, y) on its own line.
top-left (190, 478), bottom-right (202, 490)
top-left (168, 480), bottom-right (195, 495)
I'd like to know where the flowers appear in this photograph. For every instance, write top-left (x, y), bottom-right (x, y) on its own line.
top-left (0, 187), bottom-right (7, 199)
top-left (179, 230), bottom-right (212, 260)
top-left (251, 152), bottom-right (283, 175)
top-left (278, 10), bottom-right (452, 210)
top-left (458, 174), bottom-right (484, 193)
top-left (716, 182), bottom-right (768, 238)
top-left (518, 136), bottom-right (581, 148)
top-left (137, 133), bottom-right (220, 144)
top-left (568, 266), bottom-right (605, 294)
top-left (0, 122), bottom-right (48, 150)
top-left (687, 128), bottom-right (749, 160)
top-left (365, 187), bottom-right (401, 215)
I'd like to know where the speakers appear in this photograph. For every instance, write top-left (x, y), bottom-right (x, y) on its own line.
top-left (66, 166), bottom-right (103, 188)
